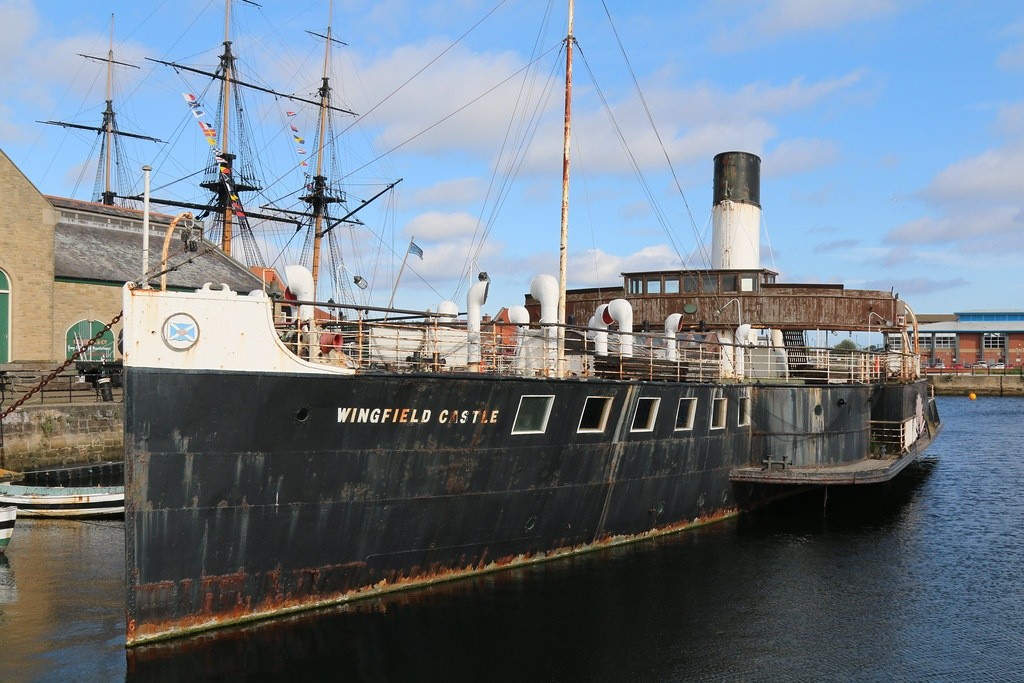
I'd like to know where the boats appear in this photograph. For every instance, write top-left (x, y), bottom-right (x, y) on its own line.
top-left (0, 505), bottom-right (22, 552)
top-left (0, 481), bottom-right (131, 517)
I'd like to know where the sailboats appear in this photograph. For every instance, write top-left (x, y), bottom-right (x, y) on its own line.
top-left (116, 0), bottom-right (943, 670)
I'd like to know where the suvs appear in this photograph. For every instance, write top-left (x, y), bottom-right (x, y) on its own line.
top-left (997, 363), bottom-right (1005, 369)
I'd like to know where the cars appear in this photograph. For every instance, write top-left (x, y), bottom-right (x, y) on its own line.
top-left (990, 363), bottom-right (1000, 369)
top-left (934, 362), bottom-right (946, 368)
top-left (978, 361), bottom-right (988, 366)
top-left (972, 362), bottom-right (983, 369)
top-left (964, 363), bottom-right (971, 368)
top-left (953, 364), bottom-right (965, 369)
top-left (1006, 364), bottom-right (1015, 369)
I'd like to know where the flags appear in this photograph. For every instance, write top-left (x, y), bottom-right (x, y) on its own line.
top-left (286, 112), bottom-right (313, 191)
top-left (183, 91), bottom-right (246, 220)
top-left (409, 242), bottom-right (423, 260)
